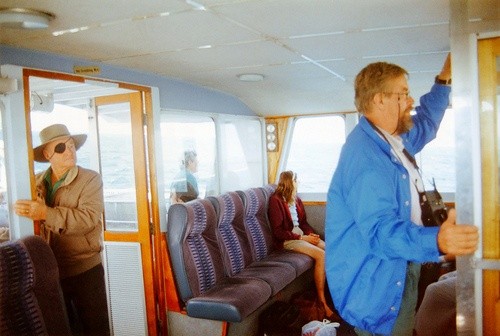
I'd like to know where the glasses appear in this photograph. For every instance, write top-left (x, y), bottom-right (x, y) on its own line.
top-left (386, 91), bottom-right (409, 100)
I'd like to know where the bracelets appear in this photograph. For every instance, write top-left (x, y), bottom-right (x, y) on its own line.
top-left (434, 75), bottom-right (451, 85)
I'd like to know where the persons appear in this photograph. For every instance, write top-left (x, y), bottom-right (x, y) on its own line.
top-left (267, 170), bottom-right (340, 322)
top-left (171, 151), bottom-right (199, 205)
top-left (14, 124), bottom-right (111, 336)
top-left (324, 51), bottom-right (479, 336)
top-left (414, 270), bottom-right (456, 336)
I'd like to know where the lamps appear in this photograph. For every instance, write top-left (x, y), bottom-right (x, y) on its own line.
top-left (0, 7), bottom-right (55, 29)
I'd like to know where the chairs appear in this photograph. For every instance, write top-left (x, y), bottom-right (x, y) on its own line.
top-left (0, 236), bottom-right (71, 336)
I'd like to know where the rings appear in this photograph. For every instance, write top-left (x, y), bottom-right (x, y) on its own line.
top-left (26, 210), bottom-right (29, 214)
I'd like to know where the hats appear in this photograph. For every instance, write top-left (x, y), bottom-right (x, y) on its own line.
top-left (32, 124), bottom-right (87, 162)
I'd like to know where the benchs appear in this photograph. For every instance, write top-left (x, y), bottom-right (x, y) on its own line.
top-left (160, 184), bottom-right (316, 336)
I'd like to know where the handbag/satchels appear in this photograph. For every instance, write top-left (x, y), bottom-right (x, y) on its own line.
top-left (302, 319), bottom-right (340, 336)
